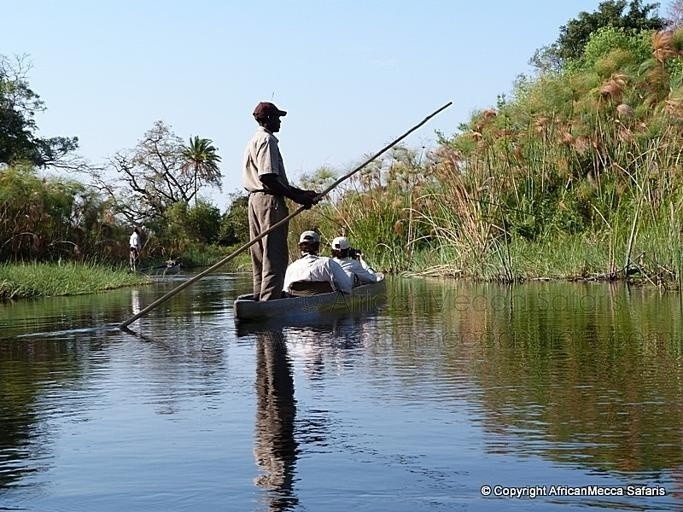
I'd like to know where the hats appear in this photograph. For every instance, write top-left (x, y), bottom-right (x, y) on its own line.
top-left (253, 101), bottom-right (287, 120)
top-left (298, 229), bottom-right (321, 246)
top-left (331, 235), bottom-right (351, 251)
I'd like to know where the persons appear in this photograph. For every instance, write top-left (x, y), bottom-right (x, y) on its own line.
top-left (330, 236), bottom-right (385, 283)
top-left (282, 230), bottom-right (354, 295)
top-left (241, 100), bottom-right (321, 301)
top-left (128, 227), bottom-right (142, 272)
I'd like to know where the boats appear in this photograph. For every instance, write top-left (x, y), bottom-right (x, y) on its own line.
top-left (233, 274), bottom-right (390, 318)
top-left (232, 310), bottom-right (381, 337)
